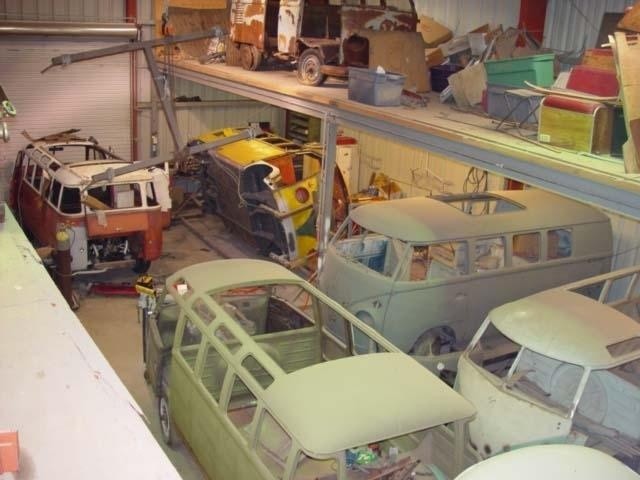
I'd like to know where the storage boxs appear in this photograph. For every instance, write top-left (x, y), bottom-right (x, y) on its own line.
top-left (350, 46), bottom-right (613, 159)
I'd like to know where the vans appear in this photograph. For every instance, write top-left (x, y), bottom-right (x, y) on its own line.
top-left (188, 123), bottom-right (350, 268)
top-left (452, 265), bottom-right (640, 459)
top-left (314, 188), bottom-right (614, 352)
top-left (230, 1), bottom-right (421, 86)
top-left (135, 257), bottom-right (476, 480)
top-left (8, 128), bottom-right (172, 283)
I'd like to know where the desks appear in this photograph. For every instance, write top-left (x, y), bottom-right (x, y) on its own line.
top-left (147, 38), bottom-right (640, 317)
